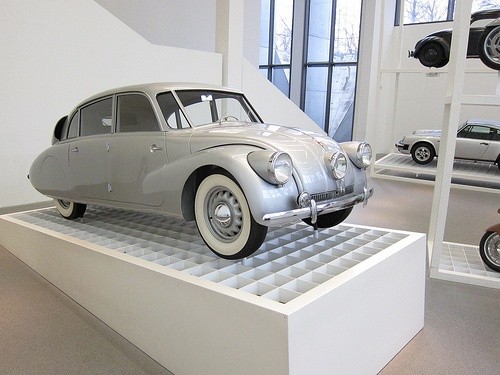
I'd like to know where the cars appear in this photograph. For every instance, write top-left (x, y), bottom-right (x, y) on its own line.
top-left (395, 118), bottom-right (500, 169)
top-left (407, 8), bottom-right (500, 72)
top-left (25, 82), bottom-right (373, 260)
